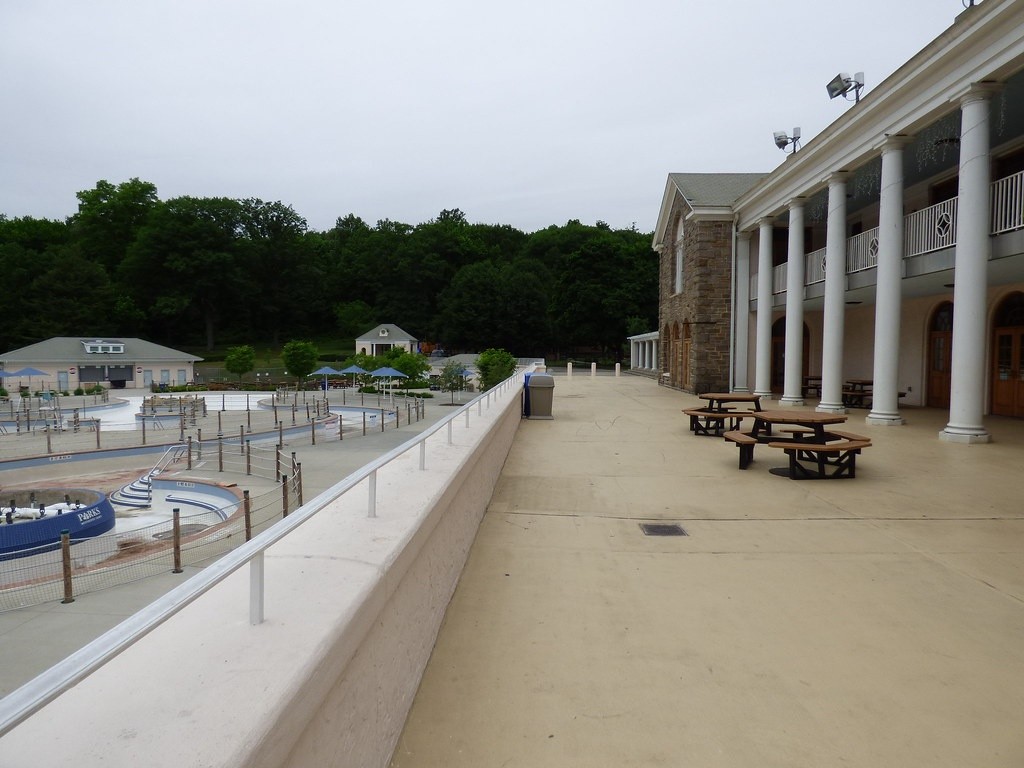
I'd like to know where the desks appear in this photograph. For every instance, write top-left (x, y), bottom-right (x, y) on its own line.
top-left (845, 379), bottom-right (873, 406)
top-left (752, 411), bottom-right (848, 478)
top-left (698, 393), bottom-right (761, 436)
top-left (803, 376), bottom-right (822, 381)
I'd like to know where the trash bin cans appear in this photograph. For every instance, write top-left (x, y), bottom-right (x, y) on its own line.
top-left (528, 374), bottom-right (555, 419)
top-left (524, 373), bottom-right (546, 415)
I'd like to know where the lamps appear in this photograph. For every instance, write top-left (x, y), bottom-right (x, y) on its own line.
top-left (773, 131), bottom-right (788, 150)
top-left (826, 73), bottom-right (852, 99)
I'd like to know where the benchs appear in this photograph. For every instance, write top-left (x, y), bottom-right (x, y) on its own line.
top-left (842, 384), bottom-right (851, 389)
top-left (810, 383), bottom-right (821, 397)
top-left (845, 389), bottom-right (872, 406)
top-left (723, 428), bottom-right (767, 470)
top-left (768, 441), bottom-right (872, 480)
top-left (898, 392), bottom-right (906, 398)
top-left (842, 392), bottom-right (873, 410)
top-left (779, 429), bottom-right (871, 467)
top-left (747, 407), bottom-right (773, 436)
top-left (681, 406), bottom-right (737, 431)
top-left (684, 410), bottom-right (759, 438)
top-left (801, 386), bottom-right (821, 399)
